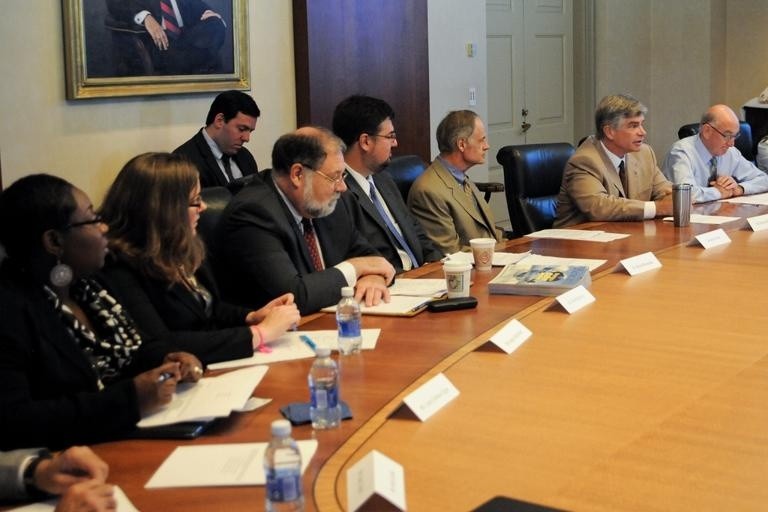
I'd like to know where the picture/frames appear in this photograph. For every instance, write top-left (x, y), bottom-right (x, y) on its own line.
top-left (61, 0), bottom-right (253, 101)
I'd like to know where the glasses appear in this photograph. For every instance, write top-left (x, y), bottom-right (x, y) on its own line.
top-left (317, 170), bottom-right (348, 185)
top-left (371, 131), bottom-right (397, 139)
top-left (61, 214), bottom-right (103, 230)
top-left (188, 196), bottom-right (202, 209)
top-left (702, 122), bottom-right (740, 141)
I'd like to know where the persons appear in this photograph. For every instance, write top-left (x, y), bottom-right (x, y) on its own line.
top-left (0, 444), bottom-right (118, 512)
top-left (95, 151), bottom-right (302, 366)
top-left (660, 103), bottom-right (768, 206)
top-left (551, 93), bottom-right (674, 230)
top-left (0, 172), bottom-right (204, 439)
top-left (105, 0), bottom-right (228, 75)
top-left (406, 108), bottom-right (505, 254)
top-left (218, 125), bottom-right (397, 317)
top-left (331, 95), bottom-right (444, 274)
top-left (171, 89), bottom-right (260, 189)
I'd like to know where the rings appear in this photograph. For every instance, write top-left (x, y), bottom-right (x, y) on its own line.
top-left (194, 366), bottom-right (203, 375)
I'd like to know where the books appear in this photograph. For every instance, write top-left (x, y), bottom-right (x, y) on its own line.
top-left (487, 262), bottom-right (593, 297)
top-left (321, 277), bottom-right (475, 318)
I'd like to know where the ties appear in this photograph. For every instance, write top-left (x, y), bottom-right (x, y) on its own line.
top-left (300, 218), bottom-right (323, 273)
top-left (464, 176), bottom-right (473, 198)
top-left (708, 158), bottom-right (717, 186)
top-left (618, 160), bottom-right (626, 193)
top-left (369, 182), bottom-right (418, 268)
top-left (159, 0), bottom-right (180, 36)
top-left (221, 154), bottom-right (235, 182)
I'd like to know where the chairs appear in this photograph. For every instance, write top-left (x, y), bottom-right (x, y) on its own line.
top-left (229, 169), bottom-right (272, 194)
top-left (677, 120), bottom-right (757, 169)
top-left (388, 156), bottom-right (427, 205)
top-left (497, 142), bottom-right (575, 236)
top-left (199, 186), bottom-right (235, 289)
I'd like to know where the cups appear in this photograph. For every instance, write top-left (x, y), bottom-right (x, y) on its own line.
top-left (443, 261), bottom-right (472, 297)
top-left (470, 237), bottom-right (496, 271)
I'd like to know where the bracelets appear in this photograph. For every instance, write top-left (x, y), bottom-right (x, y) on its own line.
top-left (251, 325), bottom-right (272, 353)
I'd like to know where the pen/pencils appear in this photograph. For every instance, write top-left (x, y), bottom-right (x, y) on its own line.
top-left (408, 301), bottom-right (426, 317)
top-left (299, 333), bottom-right (320, 355)
top-left (154, 373), bottom-right (174, 387)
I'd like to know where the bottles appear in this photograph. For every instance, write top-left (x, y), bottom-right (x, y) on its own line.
top-left (336, 287), bottom-right (363, 356)
top-left (264, 419), bottom-right (304, 512)
top-left (307, 345), bottom-right (342, 430)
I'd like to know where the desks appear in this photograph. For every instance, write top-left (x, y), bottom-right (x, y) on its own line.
top-left (0, 193), bottom-right (767, 512)
